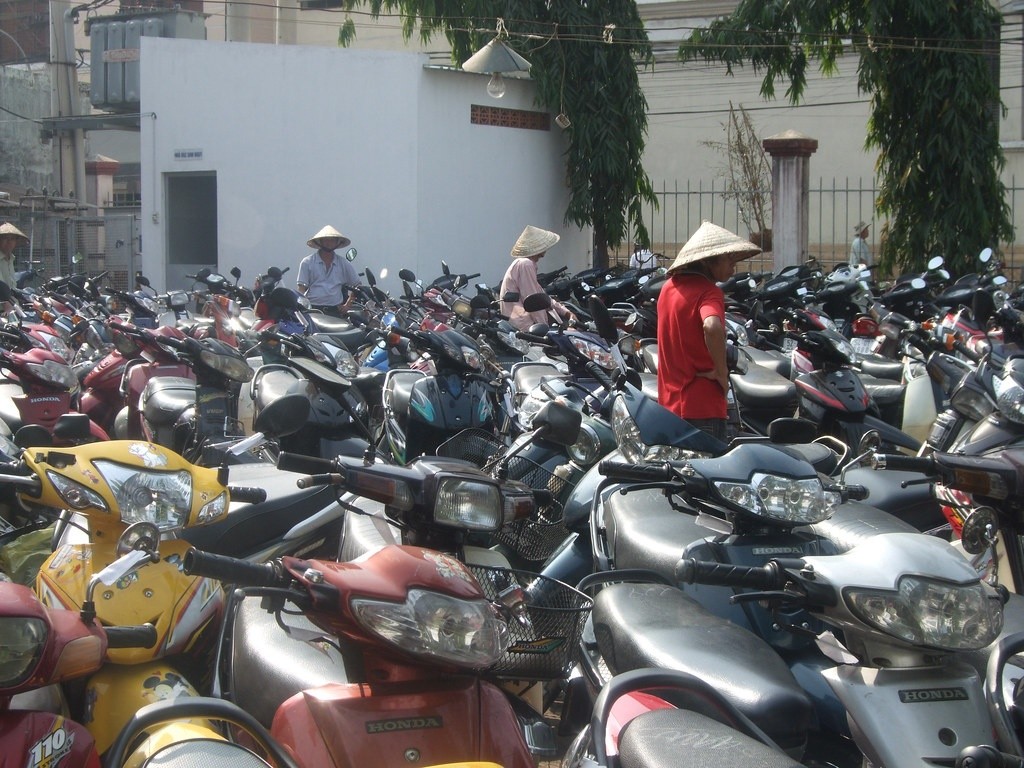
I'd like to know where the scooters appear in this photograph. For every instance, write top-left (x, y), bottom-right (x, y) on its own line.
top-left (0, 243), bottom-right (1024, 767)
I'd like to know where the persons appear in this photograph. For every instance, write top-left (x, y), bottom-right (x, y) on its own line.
top-left (629, 237), bottom-right (658, 277)
top-left (296, 225), bottom-right (363, 319)
top-left (501, 225), bottom-right (579, 332)
top-left (848, 221), bottom-right (873, 266)
top-left (657, 219), bottom-right (764, 443)
top-left (0, 221), bottom-right (30, 290)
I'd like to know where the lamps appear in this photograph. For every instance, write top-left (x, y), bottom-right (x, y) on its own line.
top-left (462, 18), bottom-right (533, 100)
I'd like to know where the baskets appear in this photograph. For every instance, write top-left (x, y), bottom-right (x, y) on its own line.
top-left (463, 565), bottom-right (595, 678)
top-left (436, 428), bottom-right (574, 561)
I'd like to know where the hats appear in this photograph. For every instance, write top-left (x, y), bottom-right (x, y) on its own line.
top-left (666, 219), bottom-right (762, 278)
top-left (850, 221), bottom-right (870, 237)
top-left (511, 225), bottom-right (559, 259)
top-left (0, 223), bottom-right (30, 247)
top-left (306, 225), bottom-right (350, 249)
